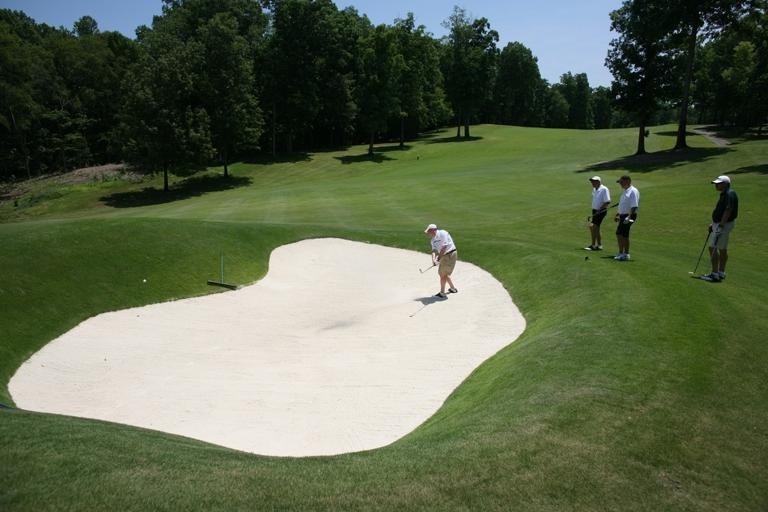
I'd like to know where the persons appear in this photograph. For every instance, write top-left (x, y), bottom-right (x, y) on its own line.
top-left (701, 174), bottom-right (737, 282)
top-left (425, 223), bottom-right (458, 298)
top-left (615, 176), bottom-right (642, 263)
top-left (586, 175), bottom-right (609, 253)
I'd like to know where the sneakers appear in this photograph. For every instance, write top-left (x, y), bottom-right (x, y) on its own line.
top-left (584, 245), bottom-right (596, 250)
top-left (623, 252), bottom-right (630, 259)
top-left (614, 254), bottom-right (624, 260)
top-left (596, 245), bottom-right (603, 250)
top-left (435, 292), bottom-right (446, 298)
top-left (700, 274), bottom-right (720, 282)
top-left (717, 271), bottom-right (725, 281)
top-left (448, 288), bottom-right (457, 293)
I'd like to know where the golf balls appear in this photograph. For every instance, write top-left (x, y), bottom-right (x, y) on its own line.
top-left (142, 279), bottom-right (146, 283)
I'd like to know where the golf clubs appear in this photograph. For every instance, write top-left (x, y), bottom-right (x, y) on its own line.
top-left (688, 231), bottom-right (711, 275)
top-left (588, 203), bottom-right (619, 222)
top-left (419, 265), bottom-right (435, 273)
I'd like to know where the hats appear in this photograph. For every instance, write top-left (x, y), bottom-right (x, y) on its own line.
top-left (589, 175), bottom-right (601, 183)
top-left (711, 174), bottom-right (731, 184)
top-left (616, 175), bottom-right (632, 183)
top-left (424, 223), bottom-right (437, 233)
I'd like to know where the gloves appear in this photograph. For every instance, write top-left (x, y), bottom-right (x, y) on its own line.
top-left (623, 216), bottom-right (631, 224)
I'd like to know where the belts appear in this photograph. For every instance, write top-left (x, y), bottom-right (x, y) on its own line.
top-left (445, 249), bottom-right (456, 255)
